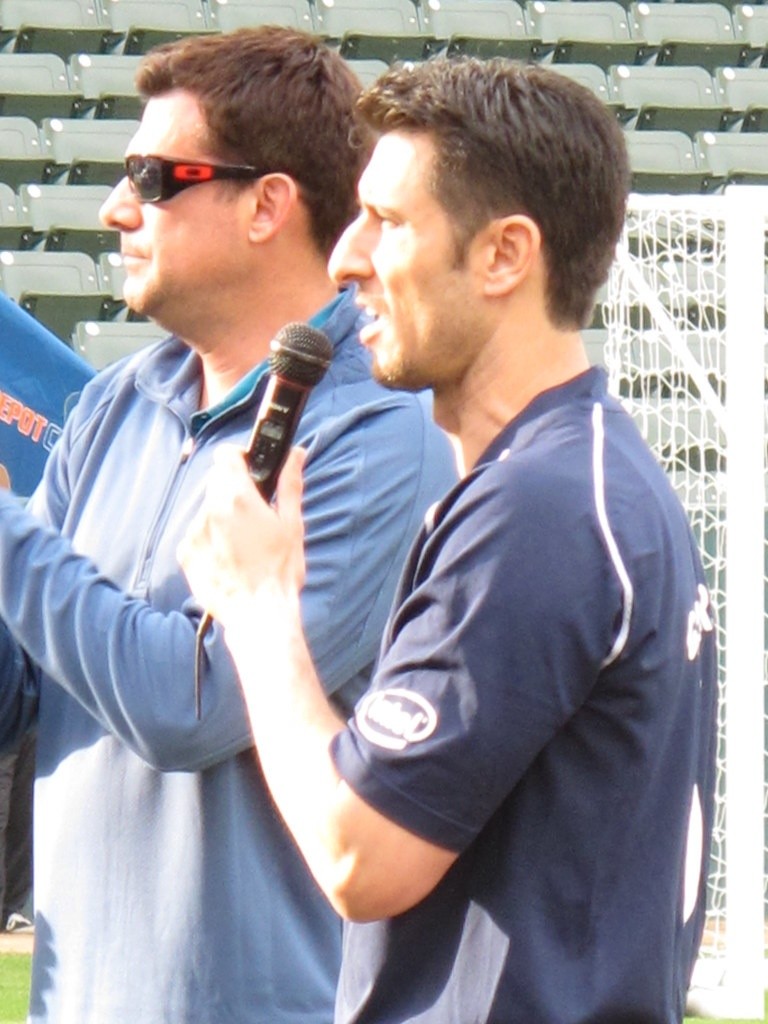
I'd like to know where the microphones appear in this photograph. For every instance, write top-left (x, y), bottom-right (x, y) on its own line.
top-left (244, 322), bottom-right (333, 506)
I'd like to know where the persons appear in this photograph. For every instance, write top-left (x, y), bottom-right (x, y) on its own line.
top-left (174, 49), bottom-right (717, 1024)
top-left (0, 24), bottom-right (459, 1023)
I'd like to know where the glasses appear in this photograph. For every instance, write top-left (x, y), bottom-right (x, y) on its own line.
top-left (124, 154), bottom-right (267, 202)
